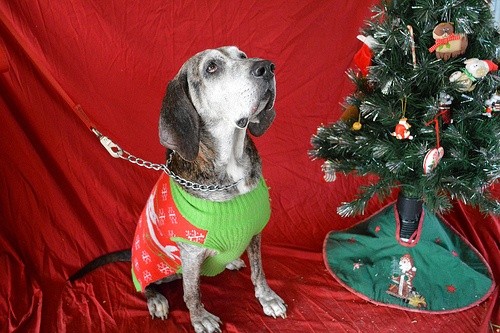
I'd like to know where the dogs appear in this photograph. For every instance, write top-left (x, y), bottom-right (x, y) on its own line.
top-left (67, 45), bottom-right (289, 333)
